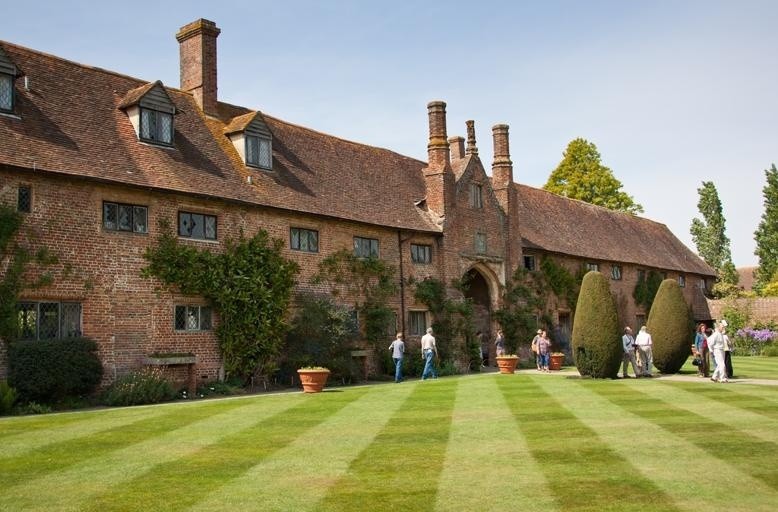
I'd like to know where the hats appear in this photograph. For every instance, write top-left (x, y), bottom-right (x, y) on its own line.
top-left (537, 329), bottom-right (543, 333)
top-left (720, 319), bottom-right (728, 327)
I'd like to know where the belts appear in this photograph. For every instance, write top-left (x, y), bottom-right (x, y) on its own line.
top-left (424, 348), bottom-right (429, 351)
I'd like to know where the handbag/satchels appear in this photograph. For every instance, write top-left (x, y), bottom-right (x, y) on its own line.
top-left (692, 354), bottom-right (704, 366)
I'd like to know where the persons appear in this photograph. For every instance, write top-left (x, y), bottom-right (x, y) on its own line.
top-left (421, 328), bottom-right (438, 380)
top-left (622, 326), bottom-right (652, 378)
top-left (495, 329), bottom-right (506, 357)
top-left (531, 329), bottom-right (551, 372)
top-left (389, 332), bottom-right (405, 383)
top-left (695, 323), bottom-right (733, 383)
top-left (476, 331), bottom-right (485, 368)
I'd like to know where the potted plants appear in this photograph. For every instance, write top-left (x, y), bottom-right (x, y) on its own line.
top-left (549, 352), bottom-right (566, 370)
top-left (496, 354), bottom-right (520, 374)
top-left (296, 365), bottom-right (331, 392)
